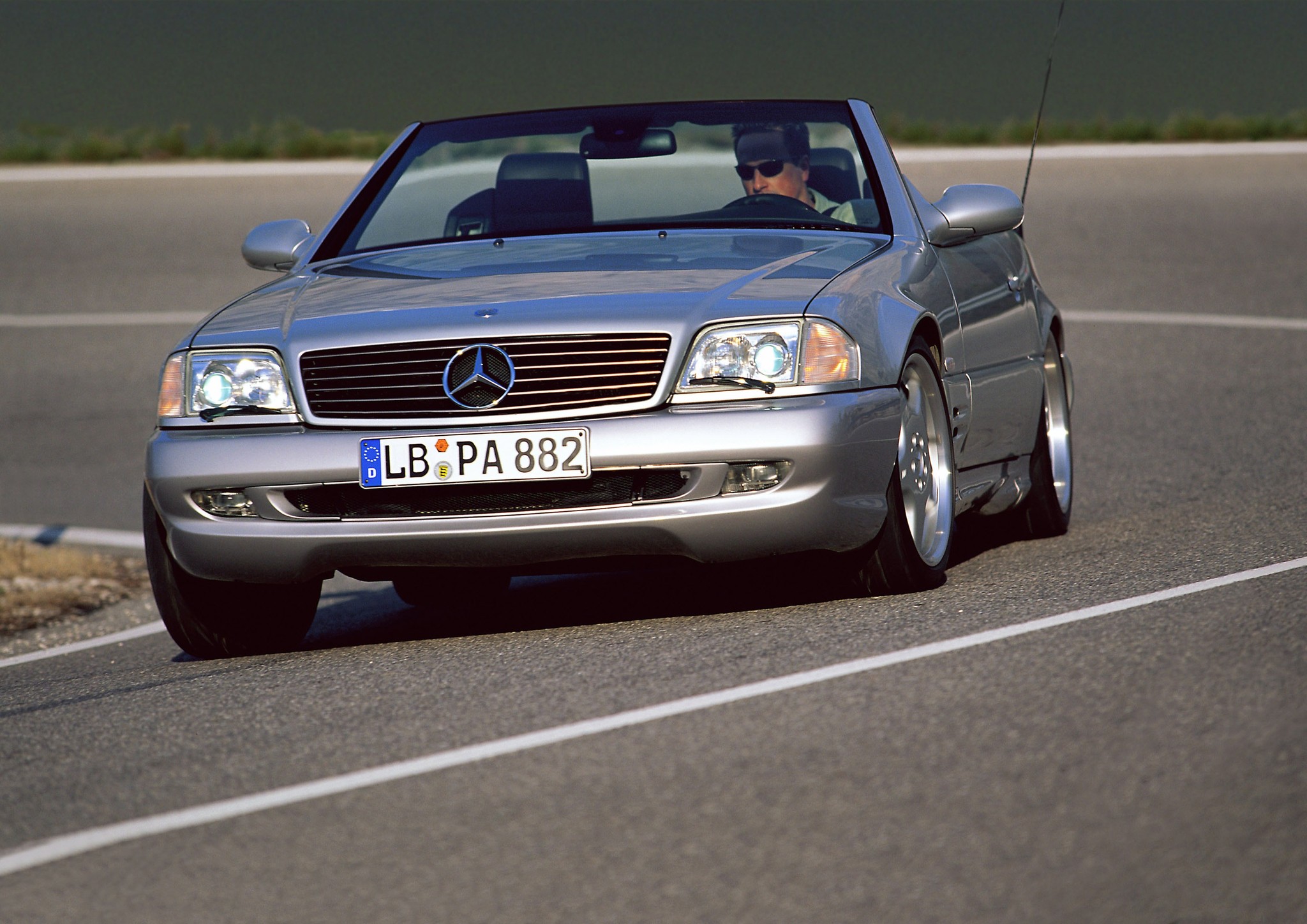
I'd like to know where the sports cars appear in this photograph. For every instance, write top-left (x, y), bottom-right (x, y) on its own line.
top-left (139, 95), bottom-right (1084, 658)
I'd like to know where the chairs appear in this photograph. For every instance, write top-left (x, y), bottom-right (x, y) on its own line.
top-left (806, 147), bottom-right (861, 204)
top-left (493, 151), bottom-right (594, 231)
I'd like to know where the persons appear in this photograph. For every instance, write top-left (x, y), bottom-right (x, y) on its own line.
top-left (729, 117), bottom-right (842, 219)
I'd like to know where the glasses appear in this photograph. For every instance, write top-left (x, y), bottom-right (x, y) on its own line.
top-left (735, 154), bottom-right (801, 181)
